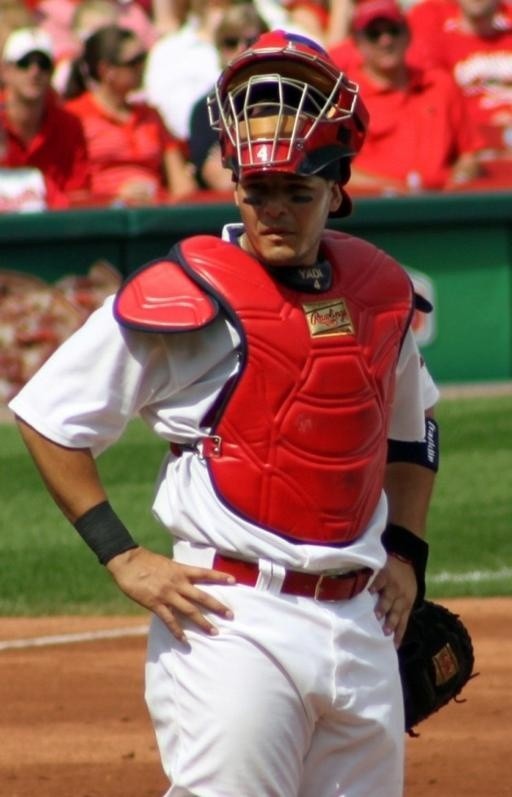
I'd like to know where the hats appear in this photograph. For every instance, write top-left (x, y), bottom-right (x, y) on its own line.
top-left (348, 0), bottom-right (407, 34)
top-left (2, 27), bottom-right (56, 65)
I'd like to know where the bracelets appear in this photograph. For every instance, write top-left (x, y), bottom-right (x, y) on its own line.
top-left (69, 501), bottom-right (138, 570)
top-left (386, 549), bottom-right (413, 565)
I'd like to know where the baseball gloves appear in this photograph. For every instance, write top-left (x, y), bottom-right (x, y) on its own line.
top-left (399, 599), bottom-right (474, 737)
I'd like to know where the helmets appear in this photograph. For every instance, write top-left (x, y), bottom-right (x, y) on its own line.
top-left (206, 28), bottom-right (369, 175)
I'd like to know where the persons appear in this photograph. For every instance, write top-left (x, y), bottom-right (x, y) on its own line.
top-left (4, 33), bottom-right (441, 797)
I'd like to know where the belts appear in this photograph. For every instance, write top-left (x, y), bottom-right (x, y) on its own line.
top-left (212, 554), bottom-right (374, 602)
